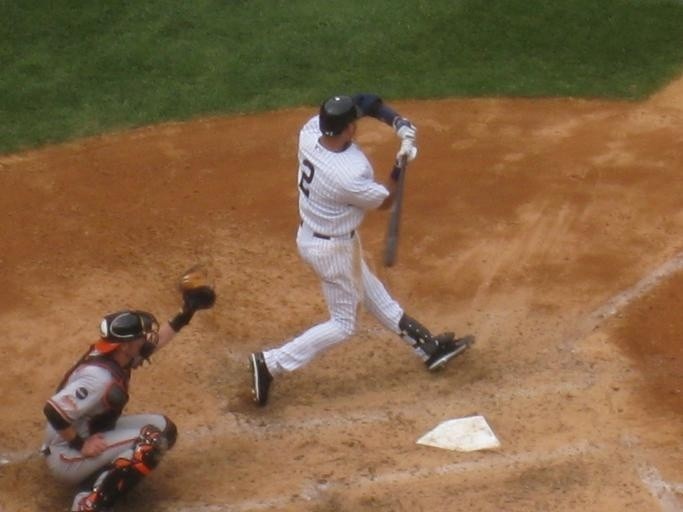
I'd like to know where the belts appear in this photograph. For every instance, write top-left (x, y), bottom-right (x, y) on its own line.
top-left (300, 221), bottom-right (354, 240)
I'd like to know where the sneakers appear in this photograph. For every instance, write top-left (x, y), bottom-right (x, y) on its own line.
top-left (249, 352), bottom-right (273, 406)
top-left (73, 491), bottom-right (96, 512)
top-left (425, 335), bottom-right (476, 372)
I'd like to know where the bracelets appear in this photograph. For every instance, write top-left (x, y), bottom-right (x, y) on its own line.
top-left (70, 431), bottom-right (85, 451)
top-left (169, 312), bottom-right (187, 334)
top-left (390, 164), bottom-right (405, 178)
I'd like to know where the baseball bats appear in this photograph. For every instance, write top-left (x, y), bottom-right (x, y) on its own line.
top-left (384, 153), bottom-right (406, 266)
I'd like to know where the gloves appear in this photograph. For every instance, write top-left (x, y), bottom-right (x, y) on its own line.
top-left (391, 143), bottom-right (417, 182)
top-left (394, 115), bottom-right (417, 142)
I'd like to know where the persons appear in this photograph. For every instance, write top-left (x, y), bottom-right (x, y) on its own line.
top-left (245, 93), bottom-right (473, 407)
top-left (34, 271), bottom-right (218, 512)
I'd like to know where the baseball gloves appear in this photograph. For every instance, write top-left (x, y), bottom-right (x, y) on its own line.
top-left (177, 267), bottom-right (215, 309)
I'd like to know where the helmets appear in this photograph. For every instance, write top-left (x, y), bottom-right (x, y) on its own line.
top-left (91, 312), bottom-right (160, 368)
top-left (319, 95), bottom-right (361, 136)
